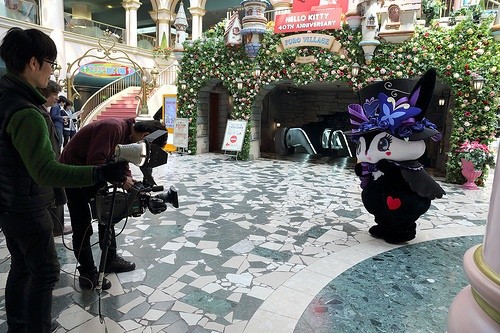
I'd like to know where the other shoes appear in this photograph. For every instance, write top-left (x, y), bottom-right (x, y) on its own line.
top-left (60, 226), bottom-right (73, 235)
top-left (50, 322), bottom-right (59, 333)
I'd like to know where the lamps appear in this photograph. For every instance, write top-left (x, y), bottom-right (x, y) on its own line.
top-left (179, 75), bottom-right (189, 89)
top-left (236, 75), bottom-right (245, 90)
top-left (274, 117), bottom-right (281, 128)
top-left (350, 58), bottom-right (366, 77)
top-left (470, 74), bottom-right (486, 93)
top-left (438, 89), bottom-right (451, 107)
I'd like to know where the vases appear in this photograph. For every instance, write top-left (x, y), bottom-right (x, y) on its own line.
top-left (461, 158), bottom-right (482, 190)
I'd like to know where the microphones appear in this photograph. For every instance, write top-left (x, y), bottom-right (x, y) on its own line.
top-left (142, 185), bottom-right (164, 192)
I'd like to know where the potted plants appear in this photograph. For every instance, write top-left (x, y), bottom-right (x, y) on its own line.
top-left (469, 4), bottom-right (483, 25)
top-left (422, 0), bottom-right (445, 27)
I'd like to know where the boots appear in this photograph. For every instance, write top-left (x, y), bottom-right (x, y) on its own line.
top-left (99, 248), bottom-right (135, 273)
top-left (76, 265), bottom-right (111, 290)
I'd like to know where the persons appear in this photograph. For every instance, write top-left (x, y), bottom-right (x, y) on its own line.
top-left (34, 81), bottom-right (168, 292)
top-left (0, 27), bottom-right (133, 333)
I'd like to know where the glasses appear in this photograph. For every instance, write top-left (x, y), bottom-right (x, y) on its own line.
top-left (36, 58), bottom-right (57, 71)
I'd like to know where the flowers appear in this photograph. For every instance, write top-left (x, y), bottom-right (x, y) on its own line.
top-left (176, 4), bottom-right (500, 186)
